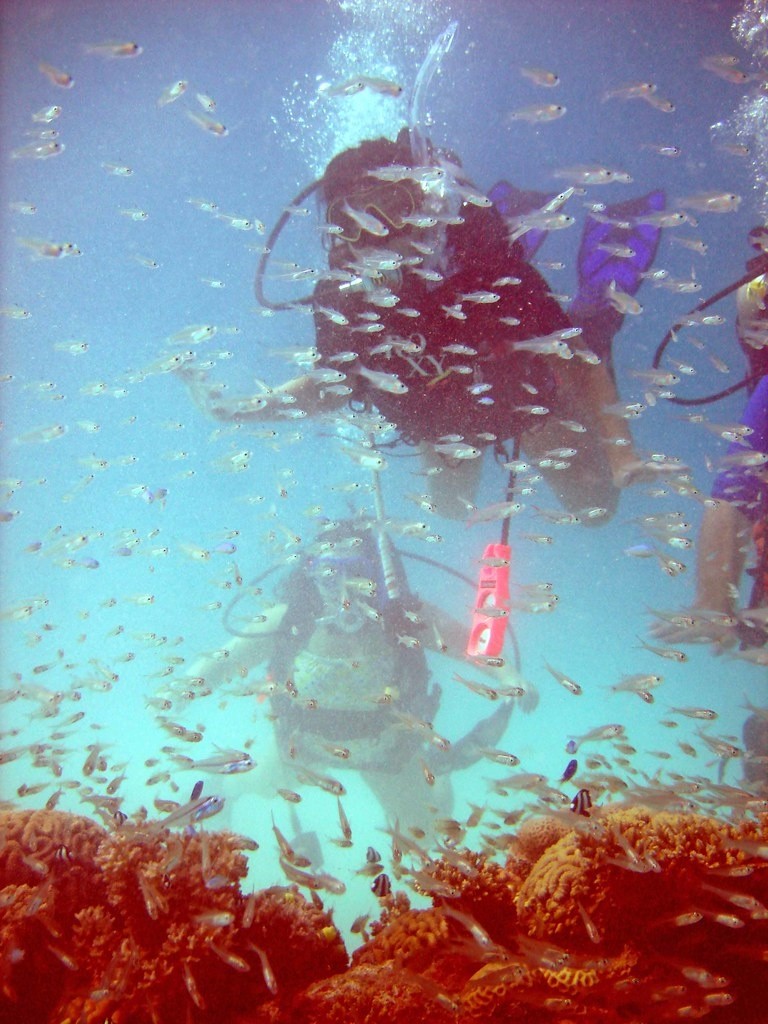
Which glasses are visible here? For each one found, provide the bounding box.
[326,180,417,243]
[311,557,373,588]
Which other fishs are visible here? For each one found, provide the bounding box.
[0,41,768,1024]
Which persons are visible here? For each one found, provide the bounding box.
[644,231,768,667]
[183,521,538,855]
[226,129,664,528]
